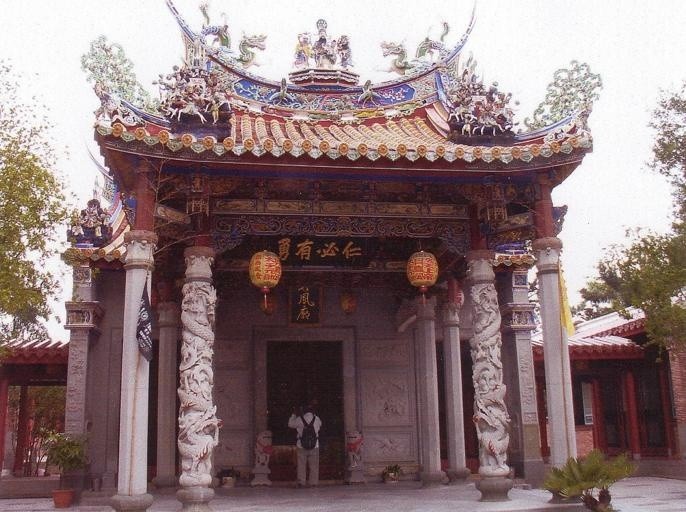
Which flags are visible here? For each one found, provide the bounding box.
[136,279,155,362]
[557,262,575,337]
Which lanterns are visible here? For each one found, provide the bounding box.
[406,252,438,308]
[249,250,283,309]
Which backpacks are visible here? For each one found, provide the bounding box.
[299,416,318,451]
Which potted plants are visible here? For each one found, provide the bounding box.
[382,464,401,484]
[42,432,91,508]
[544,449,639,512]
[215,468,241,488]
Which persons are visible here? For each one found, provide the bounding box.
[151,57,234,126]
[445,69,515,136]
[291,18,354,72]
[288,401,323,489]
